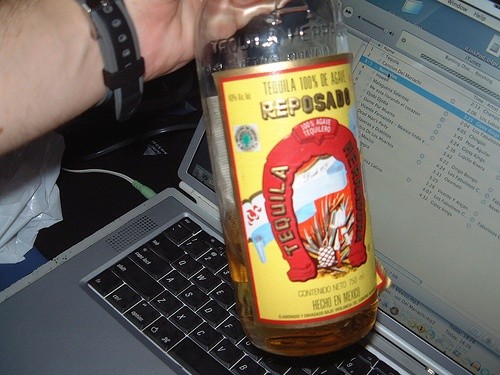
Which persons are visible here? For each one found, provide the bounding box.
[0,0,288,155]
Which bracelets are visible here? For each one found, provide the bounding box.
[81,0,146,118]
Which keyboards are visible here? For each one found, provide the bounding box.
[1,1,500,375]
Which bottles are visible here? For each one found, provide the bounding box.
[193,1,377,357]
